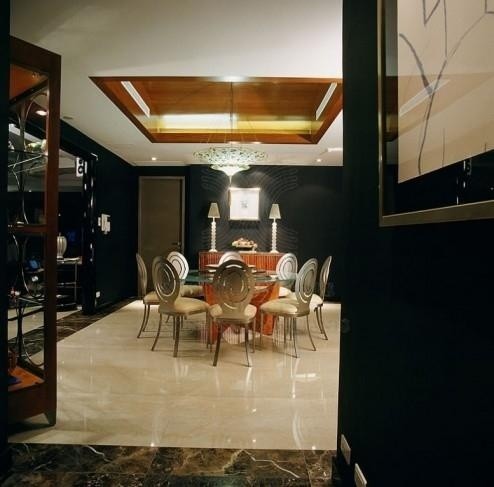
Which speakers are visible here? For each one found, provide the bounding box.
[75,158,88,178]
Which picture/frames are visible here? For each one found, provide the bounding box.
[227,187,261,221]
[376,0,494,228]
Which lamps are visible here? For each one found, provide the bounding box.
[268,203,281,253]
[208,202,220,252]
[192,82,267,186]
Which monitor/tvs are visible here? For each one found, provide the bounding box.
[27,259,40,269]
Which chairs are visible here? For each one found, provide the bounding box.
[134,251,332,366]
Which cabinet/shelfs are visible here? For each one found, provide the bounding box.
[6,32,61,424]
